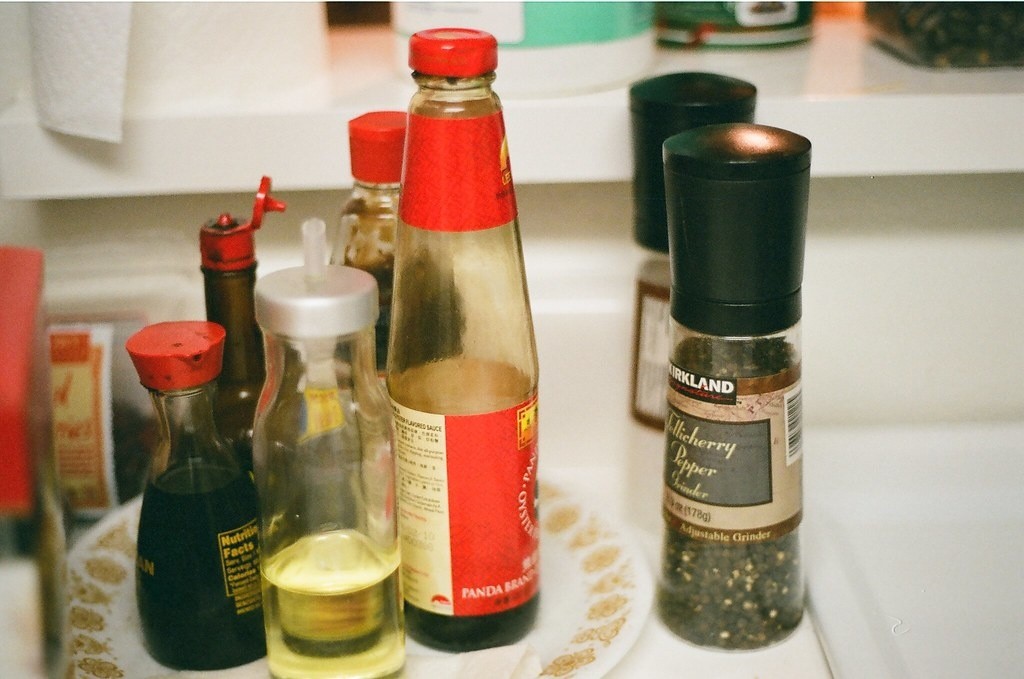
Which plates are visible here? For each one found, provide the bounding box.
[67,463,656,679]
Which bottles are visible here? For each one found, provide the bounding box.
[624,72,757,529]
[127,28,539,679]
[656,124,811,650]
[0,244,61,679]
